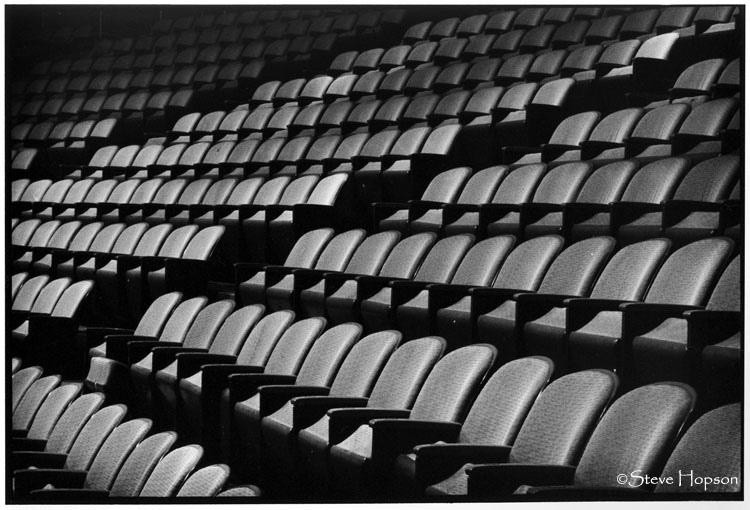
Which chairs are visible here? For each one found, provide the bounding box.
[6,4,746,503]
